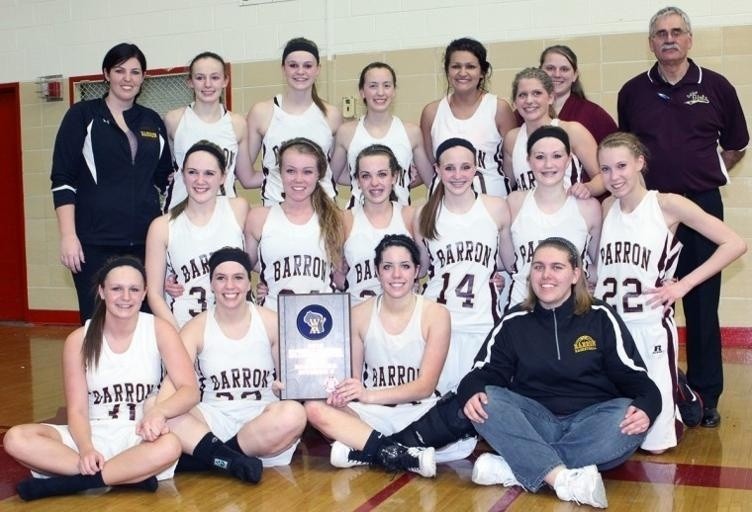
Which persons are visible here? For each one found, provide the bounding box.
[50,43,174,326]
[494,125,603,285]
[502,67,609,198]
[162,51,265,214]
[408,37,517,197]
[3,254,201,502]
[136,246,307,484]
[417,139,517,276]
[246,37,352,186]
[330,62,435,188]
[457,236,662,508]
[592,132,747,455]
[617,6,750,427]
[539,45,617,145]
[304,233,477,477]
[244,137,345,311]
[145,140,251,335]
[334,145,417,300]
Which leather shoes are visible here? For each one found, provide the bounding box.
[700,407,721,428]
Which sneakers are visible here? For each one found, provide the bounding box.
[212,445,262,483]
[553,464,608,509]
[380,442,437,478]
[471,454,528,492]
[676,367,704,429]
[329,441,375,468]
